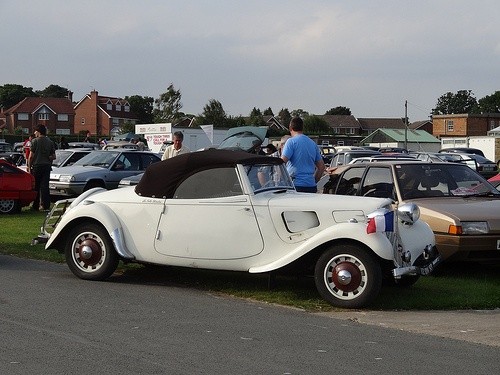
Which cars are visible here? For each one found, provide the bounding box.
[0,159,37,214]
[48,149,161,197]
[317,161,500,266]
[1,126,500,198]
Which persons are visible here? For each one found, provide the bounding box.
[280,116,325,193]
[256,135,292,187]
[27,124,55,210]
[23,134,34,171]
[160,131,189,162]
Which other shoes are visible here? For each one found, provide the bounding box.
[39,207,51,212]
[27,206,40,211]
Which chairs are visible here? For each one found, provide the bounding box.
[418,175,445,196]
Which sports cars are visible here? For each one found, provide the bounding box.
[29,148,441,310]
